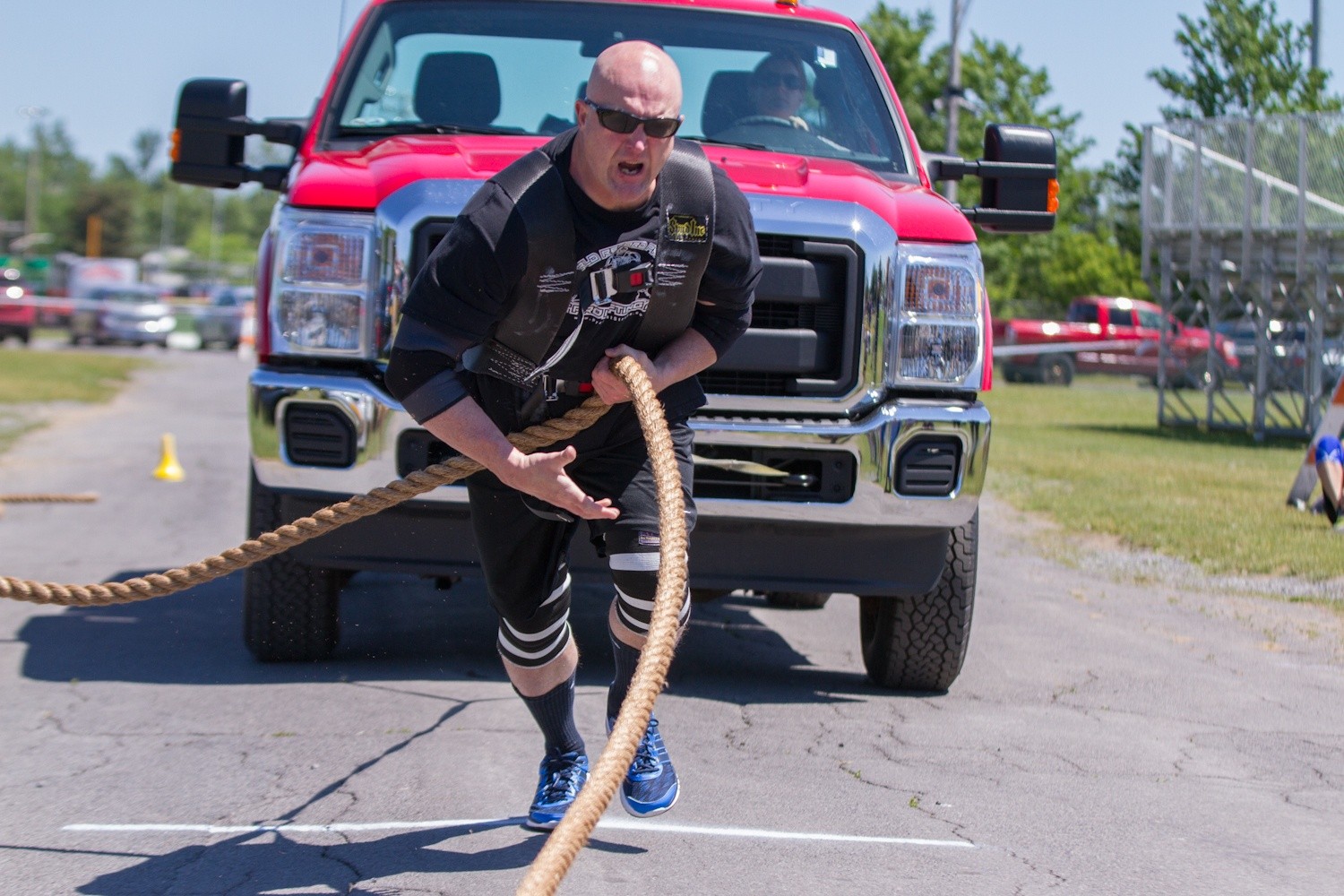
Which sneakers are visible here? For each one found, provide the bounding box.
[525,748,589,830]
[606,681,681,819]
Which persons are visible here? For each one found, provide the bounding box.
[750,49,818,135]
[1317,435,1344,524]
[387,40,764,828]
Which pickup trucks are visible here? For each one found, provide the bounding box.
[998,294,1242,394]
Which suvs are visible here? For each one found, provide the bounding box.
[165,0,1059,692]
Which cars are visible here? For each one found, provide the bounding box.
[1216,320,1340,392]
[0,245,256,352]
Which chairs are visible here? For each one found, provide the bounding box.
[700,69,756,137]
[413,50,523,132]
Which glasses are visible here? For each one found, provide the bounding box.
[757,71,802,89]
[584,97,681,137]
